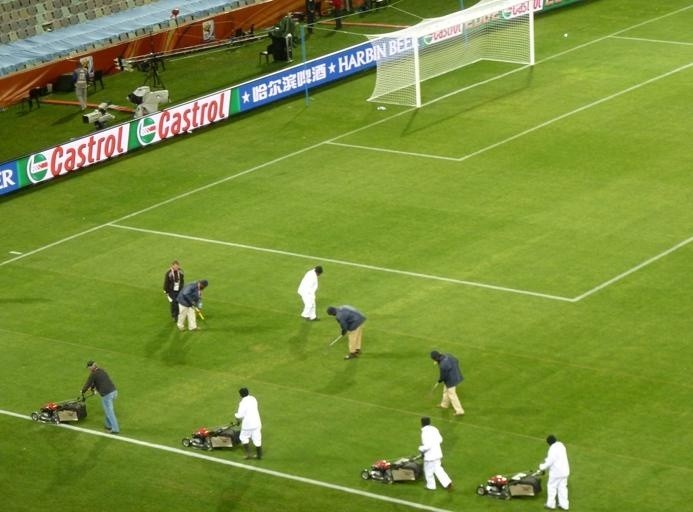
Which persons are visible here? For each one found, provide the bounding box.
[538,433,570,510]
[326,305,368,360]
[417,415,452,492]
[163,260,184,322]
[234,386,263,460]
[70,61,90,112]
[296,264,324,320]
[176,279,210,330]
[81,359,121,435]
[265,0,394,63]
[429,350,466,416]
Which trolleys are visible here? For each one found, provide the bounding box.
[31,389,97,424]
[476,468,545,501]
[182,420,240,452]
[359,451,424,484]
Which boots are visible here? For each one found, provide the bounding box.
[240,443,262,460]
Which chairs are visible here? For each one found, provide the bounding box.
[0,0,257,77]
[87,70,105,92]
[21,87,41,112]
[259,44,277,64]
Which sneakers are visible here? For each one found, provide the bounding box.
[435,401,464,418]
[344,350,362,360]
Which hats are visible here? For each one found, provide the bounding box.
[86,360,95,369]
